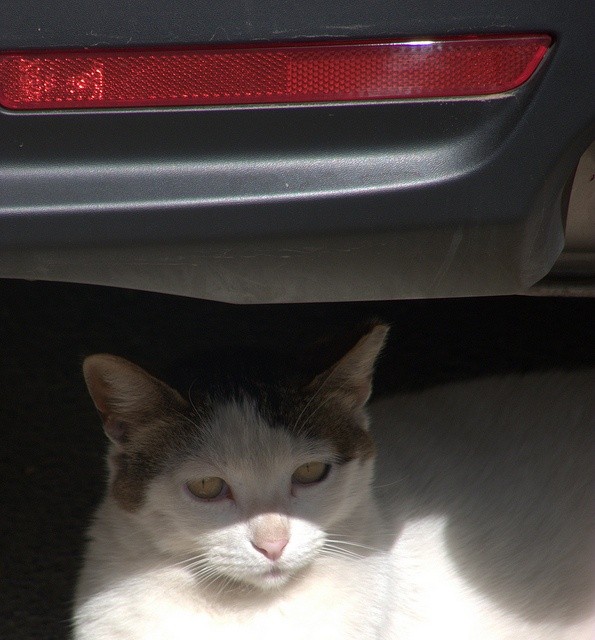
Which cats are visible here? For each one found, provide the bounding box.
[69,321,595,639]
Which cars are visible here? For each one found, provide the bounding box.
[0,0,595,321]
